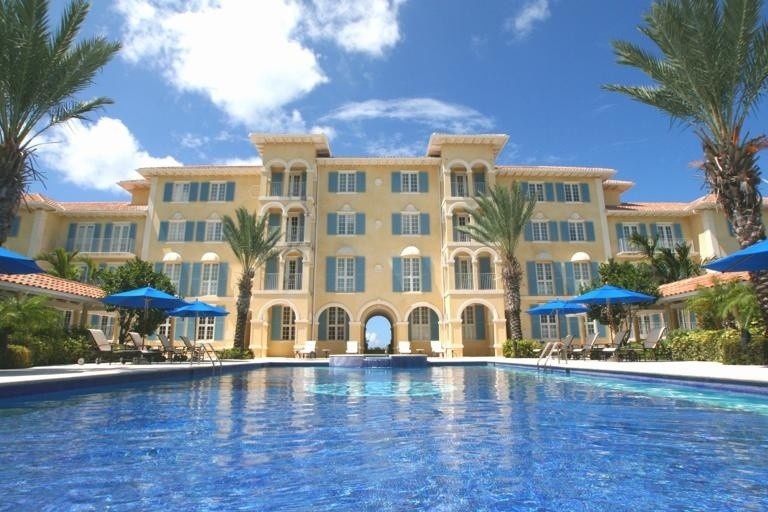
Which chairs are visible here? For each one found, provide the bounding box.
[345,340,359,354]
[398,338,445,358]
[550,326,670,362]
[83,328,206,365]
[298,339,317,358]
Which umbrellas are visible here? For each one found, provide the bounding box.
[165,297,231,361]
[564,280,658,362]
[526,297,593,357]
[0,246,48,276]
[100,283,194,364]
[699,238,768,274]
